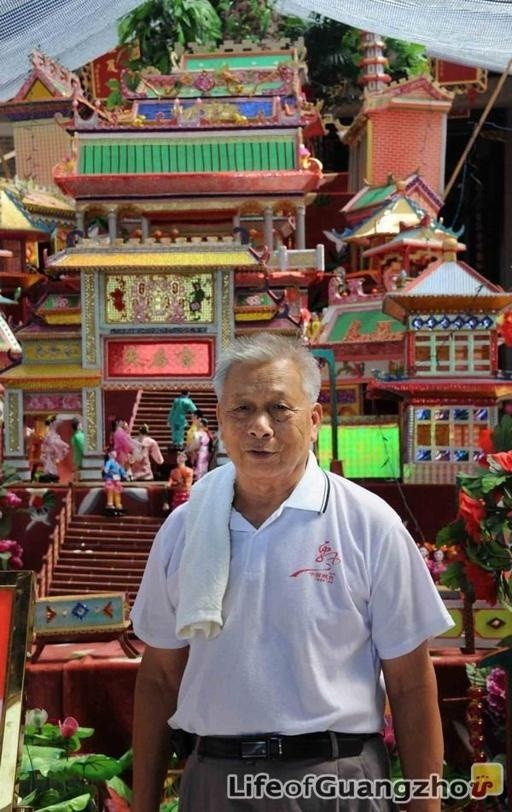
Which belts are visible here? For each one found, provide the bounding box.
[189,732,363,760]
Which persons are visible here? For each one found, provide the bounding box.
[26,390,214,518]
[125,329,456,810]
